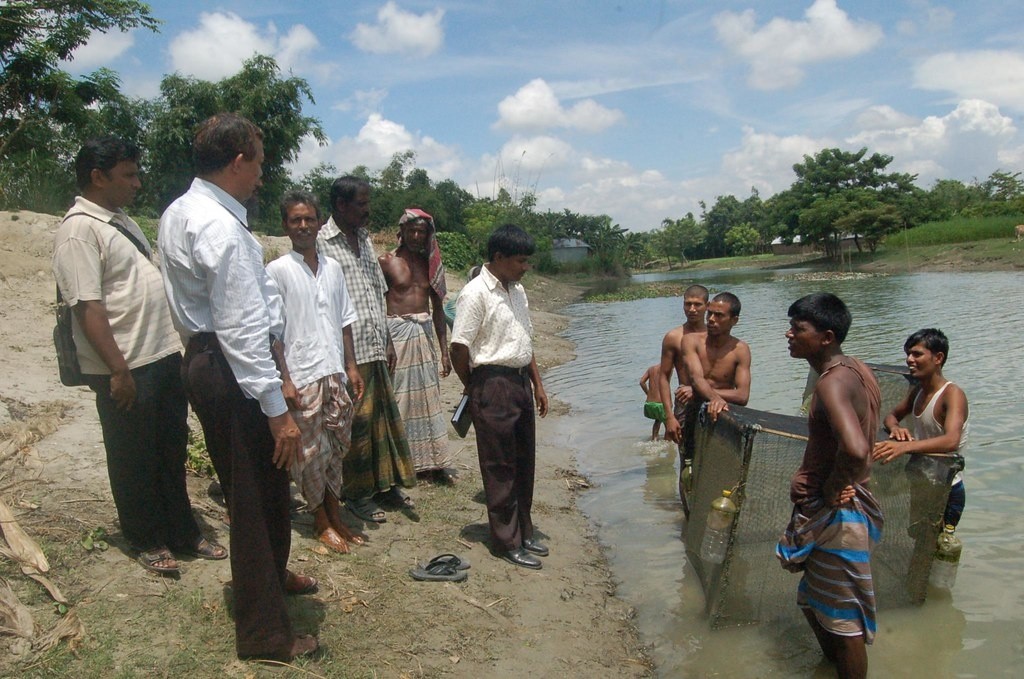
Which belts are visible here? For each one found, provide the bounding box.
[483,365,530,376]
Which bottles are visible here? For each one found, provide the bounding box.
[681,458,693,523]
[699,490,736,565]
[930,523,962,591]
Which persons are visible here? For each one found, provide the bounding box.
[50,137,229,573]
[873,328,968,540]
[261,188,369,556]
[157,112,323,665]
[639,363,673,442]
[448,224,550,570]
[773,290,884,679]
[675,292,751,463]
[376,207,455,482]
[658,285,711,522]
[315,173,418,521]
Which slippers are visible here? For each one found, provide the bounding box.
[416,554,470,569]
[352,500,386,523]
[381,486,415,510]
[409,560,467,582]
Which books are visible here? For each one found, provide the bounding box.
[451,394,472,438]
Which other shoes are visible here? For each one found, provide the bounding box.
[290,637,319,657]
[286,569,317,594]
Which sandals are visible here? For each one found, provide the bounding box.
[184,534,228,560]
[127,543,180,573]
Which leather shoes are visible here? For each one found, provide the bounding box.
[523,538,549,557]
[489,545,542,570]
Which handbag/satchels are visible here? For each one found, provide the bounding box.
[52,306,85,387]
